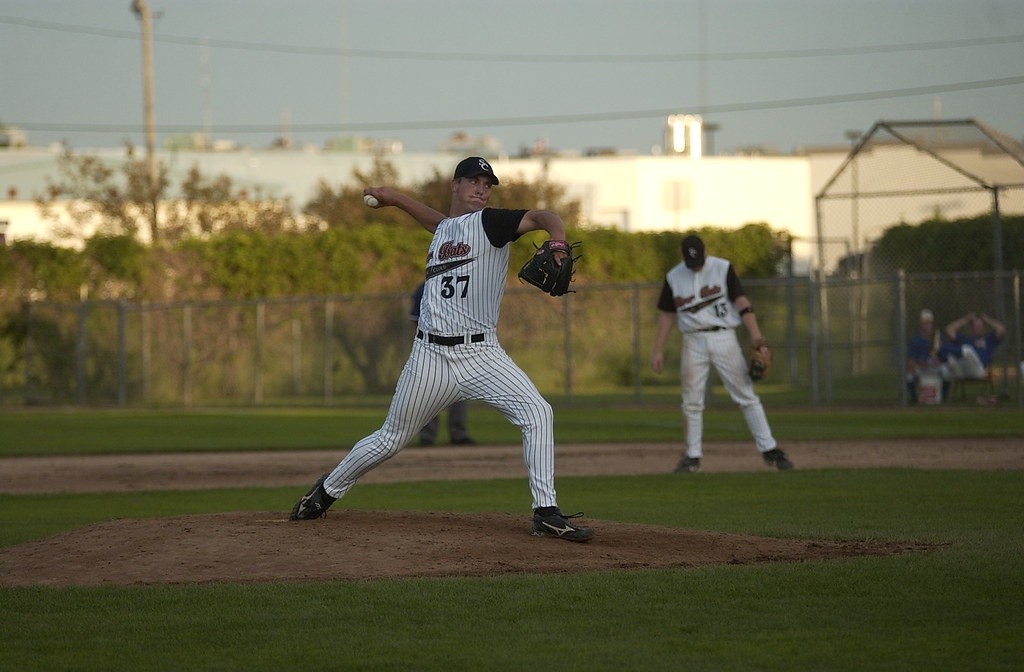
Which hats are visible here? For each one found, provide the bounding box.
[682,236,705,267]
[454,157,499,185]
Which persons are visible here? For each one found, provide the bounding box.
[907,310,1009,402]
[407,281,479,446]
[649,234,795,469]
[289,157,595,543]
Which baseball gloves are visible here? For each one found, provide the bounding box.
[517,238,584,298]
[748,336,772,383]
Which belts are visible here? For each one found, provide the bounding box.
[417,330,484,346]
[699,327,726,331]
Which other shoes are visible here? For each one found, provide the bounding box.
[763,447,793,470]
[674,455,700,474]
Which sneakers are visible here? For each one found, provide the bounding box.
[290,473,335,522]
[530,506,593,542]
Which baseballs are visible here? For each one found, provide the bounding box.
[363,194,379,208]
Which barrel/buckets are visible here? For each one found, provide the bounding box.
[918,376,942,403]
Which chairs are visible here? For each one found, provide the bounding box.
[954,354,995,401]
